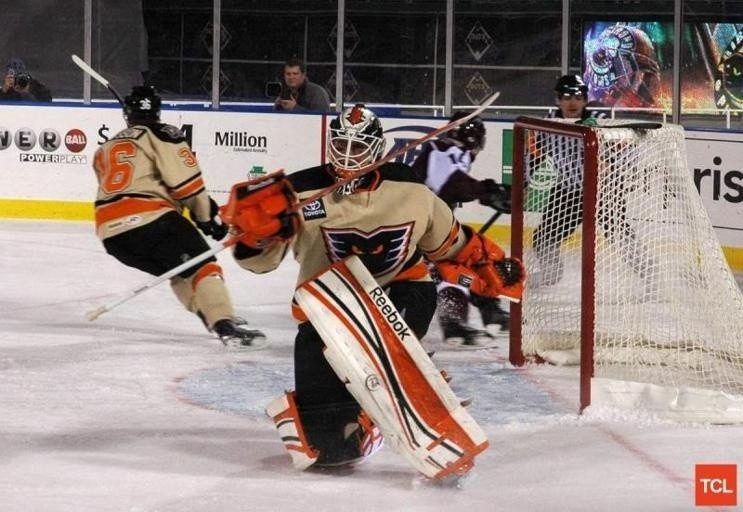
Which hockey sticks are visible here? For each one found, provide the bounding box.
[86,92,501,323]
[71,56,123,105]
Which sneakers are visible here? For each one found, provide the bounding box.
[480,308,510,330]
[442,321,496,346]
[196,308,265,347]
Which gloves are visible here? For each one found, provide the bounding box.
[190,196,227,240]
[480,178,512,215]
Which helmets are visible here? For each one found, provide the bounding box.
[122,85,161,120]
[327,102,386,197]
[555,74,589,91]
[447,109,487,158]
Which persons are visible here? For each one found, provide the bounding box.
[411,110,528,342]
[526,73,665,305]
[271,61,331,114]
[217,102,527,477]
[0,59,53,102]
[91,83,268,344]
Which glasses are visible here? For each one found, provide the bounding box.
[564,92,585,101]
